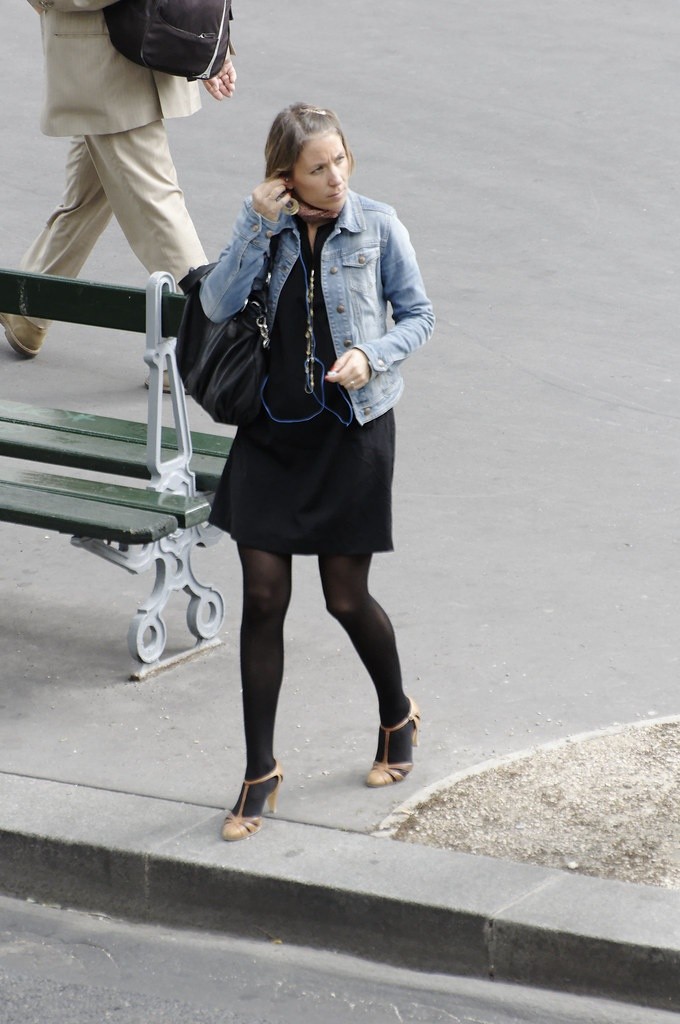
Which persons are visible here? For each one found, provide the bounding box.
[1,0,237,359]
[201,103,433,841]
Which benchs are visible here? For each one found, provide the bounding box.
[1,267,240,679]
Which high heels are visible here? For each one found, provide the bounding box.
[224,760,282,841]
[367,695,420,786]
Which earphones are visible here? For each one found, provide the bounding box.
[285,178,289,182]
[327,371,338,376]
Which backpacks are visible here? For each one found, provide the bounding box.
[103,0,236,82]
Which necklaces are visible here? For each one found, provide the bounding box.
[304,269,315,392]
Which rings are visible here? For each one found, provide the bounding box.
[269,194,276,201]
[350,381,354,388]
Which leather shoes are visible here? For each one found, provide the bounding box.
[145,370,191,394]
[0,312,44,357]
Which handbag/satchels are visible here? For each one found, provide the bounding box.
[173,233,279,425]
